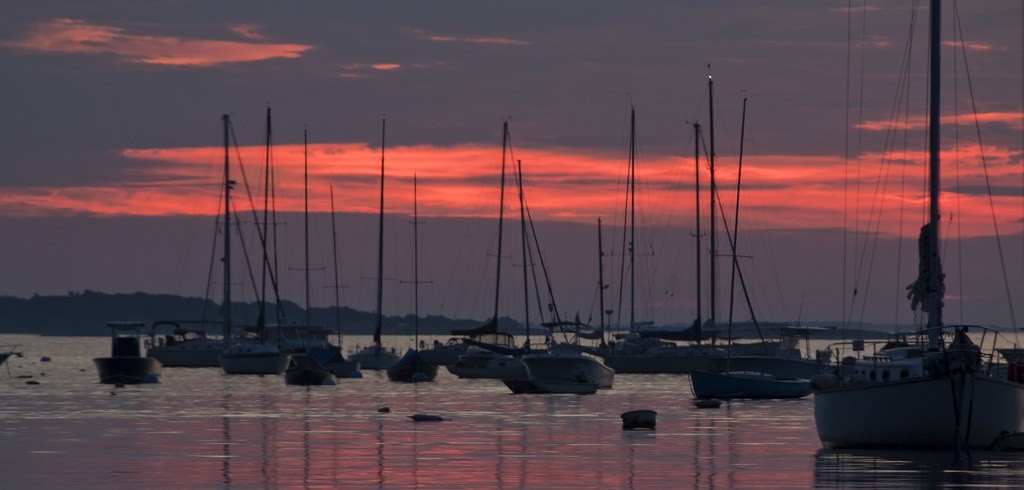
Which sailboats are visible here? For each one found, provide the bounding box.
[147,3,1024,459]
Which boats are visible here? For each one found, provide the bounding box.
[93,317,165,386]
